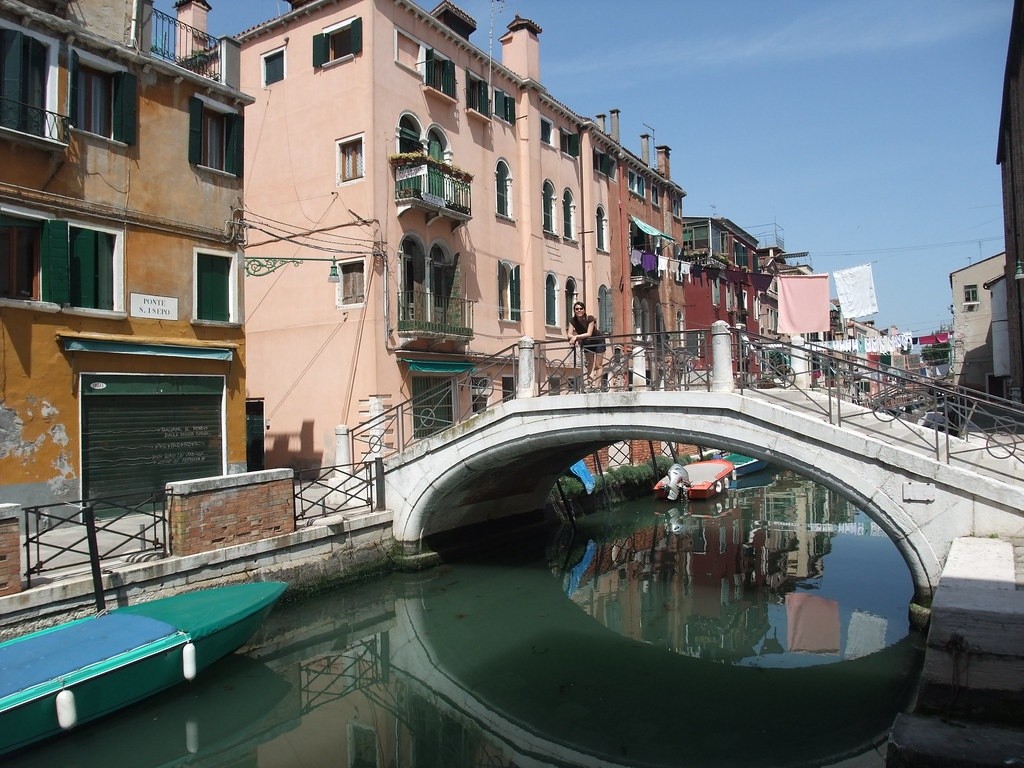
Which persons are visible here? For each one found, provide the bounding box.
[567,302,606,393]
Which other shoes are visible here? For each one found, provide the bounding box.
[594,388,600,393]
[583,386,594,393]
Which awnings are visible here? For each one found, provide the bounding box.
[631,215,676,255]
[403,358,476,373]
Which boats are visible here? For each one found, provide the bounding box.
[0,580,289,754]
[653,459,737,499]
[723,454,768,476]
[654,493,737,534]
[0,651,294,767]
[917,412,944,431]
[727,470,778,491]
